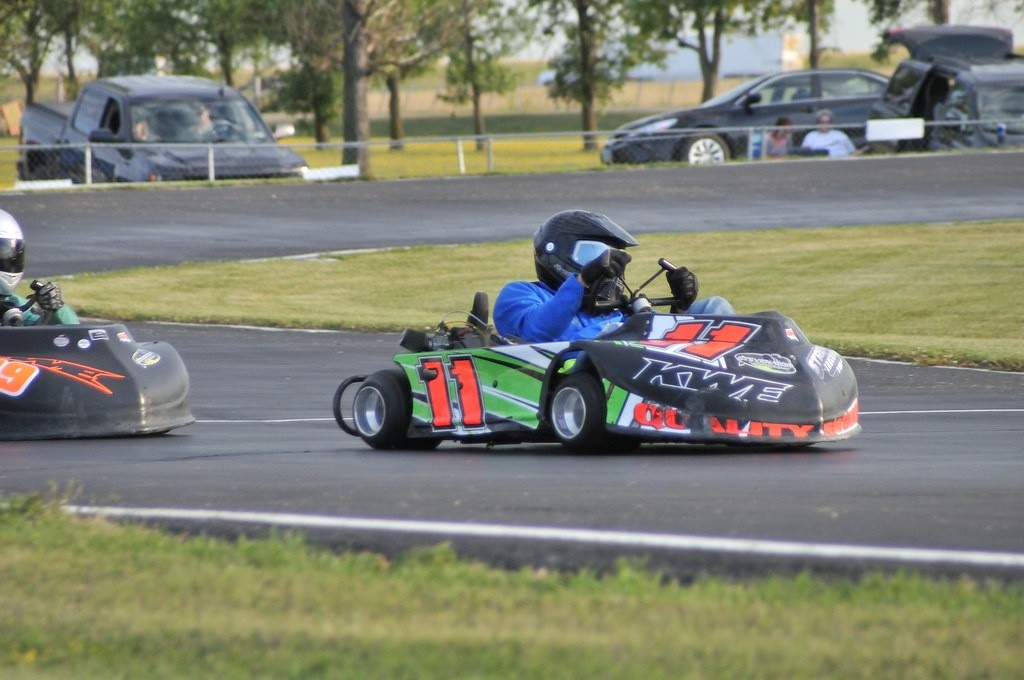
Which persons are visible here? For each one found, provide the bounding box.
[492,209,736,344]
[0,209,81,326]
[189,103,214,136]
[766,118,794,158]
[135,116,150,139]
[801,109,856,157]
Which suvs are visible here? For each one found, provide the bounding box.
[868,24,1024,153]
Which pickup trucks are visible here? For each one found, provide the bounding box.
[16,76,309,183]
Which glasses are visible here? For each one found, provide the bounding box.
[197,108,207,116]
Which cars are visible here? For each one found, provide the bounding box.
[601,67,890,166]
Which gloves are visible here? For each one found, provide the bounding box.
[665,266,699,312]
[581,248,633,287]
[36,281,65,312]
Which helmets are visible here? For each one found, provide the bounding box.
[532,209,640,311]
[0,208,25,298]
